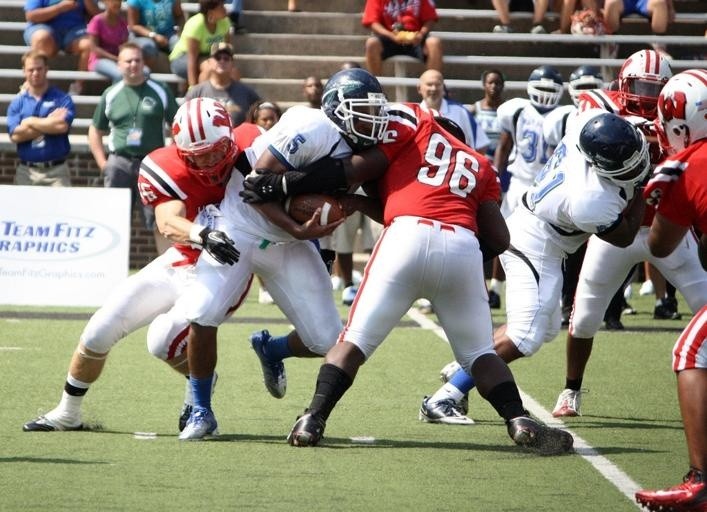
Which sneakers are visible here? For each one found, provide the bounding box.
[23,414,83,431]
[179,370,218,440]
[508,389,580,455]
[606,279,681,329]
[419,360,476,424]
[635,468,707,512]
[248,330,327,446]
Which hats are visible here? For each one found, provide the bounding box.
[210,41,233,55]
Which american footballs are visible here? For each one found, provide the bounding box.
[284,192,341,225]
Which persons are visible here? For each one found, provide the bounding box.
[22,50,707,511]
[362,0,442,78]
[492,0,677,36]
[7,0,265,232]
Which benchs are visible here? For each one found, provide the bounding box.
[0,1,707,157]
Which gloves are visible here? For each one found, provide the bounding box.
[239,168,285,204]
[199,226,241,265]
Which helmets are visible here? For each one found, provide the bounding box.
[527,50,707,187]
[171,97,239,187]
[320,67,389,152]
